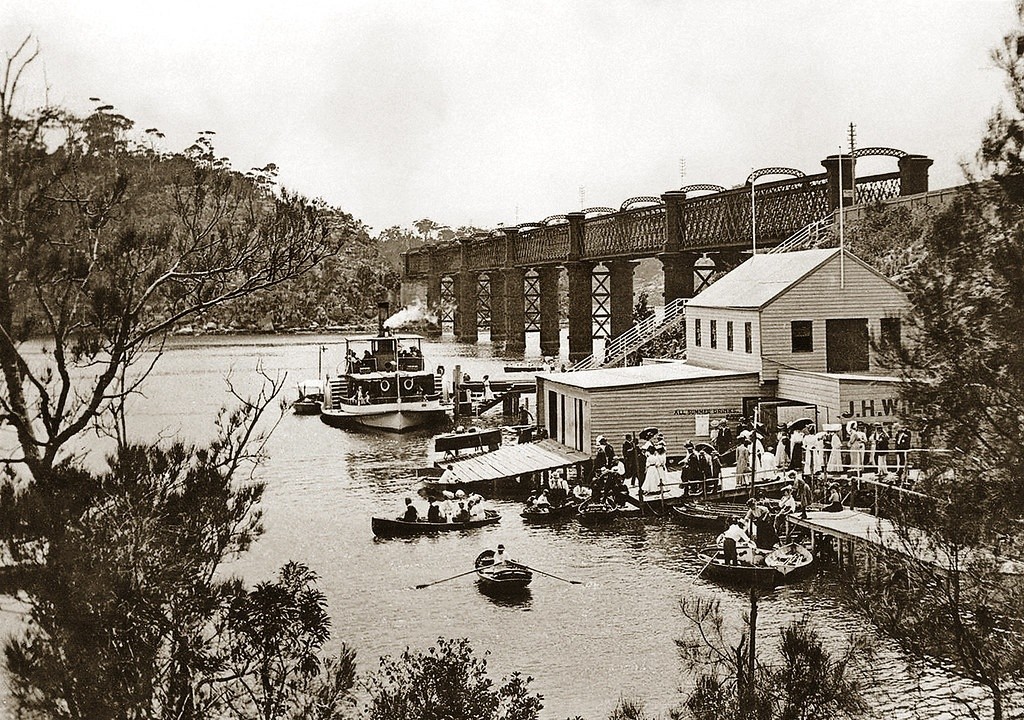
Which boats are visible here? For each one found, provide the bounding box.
[474,549,533,588]
[578,496,618,523]
[672,501,813,588]
[320,332,455,430]
[519,492,590,520]
[371,507,503,538]
[291,379,325,413]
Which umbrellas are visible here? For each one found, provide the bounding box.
[751,421,770,436]
[788,417,814,431]
[639,428,658,440]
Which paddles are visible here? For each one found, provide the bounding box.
[415,562,503,589]
[691,550,719,584]
[505,559,582,584]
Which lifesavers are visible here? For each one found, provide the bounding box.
[384,361,392,372]
[404,379,413,390]
[380,380,390,392]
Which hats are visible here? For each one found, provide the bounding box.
[776,422,787,429]
[611,455,620,462]
[496,544,505,550]
[719,418,728,423]
[787,470,797,476]
[710,420,719,427]
[805,424,814,431]
[738,518,748,526]
[828,481,840,490]
[455,490,465,498]
[746,498,756,506]
[736,435,747,439]
[442,490,454,499]
[781,484,792,491]
[857,423,864,428]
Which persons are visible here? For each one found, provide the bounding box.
[723,497,780,566]
[397,343,422,358]
[525,432,671,513]
[344,349,372,364]
[438,465,459,483]
[481,375,496,403]
[323,374,333,409]
[396,490,486,522]
[463,373,470,383]
[518,406,534,425]
[773,470,844,535]
[680,415,779,498]
[355,385,371,406]
[493,544,511,567]
[775,421,934,476]
[452,423,482,434]
